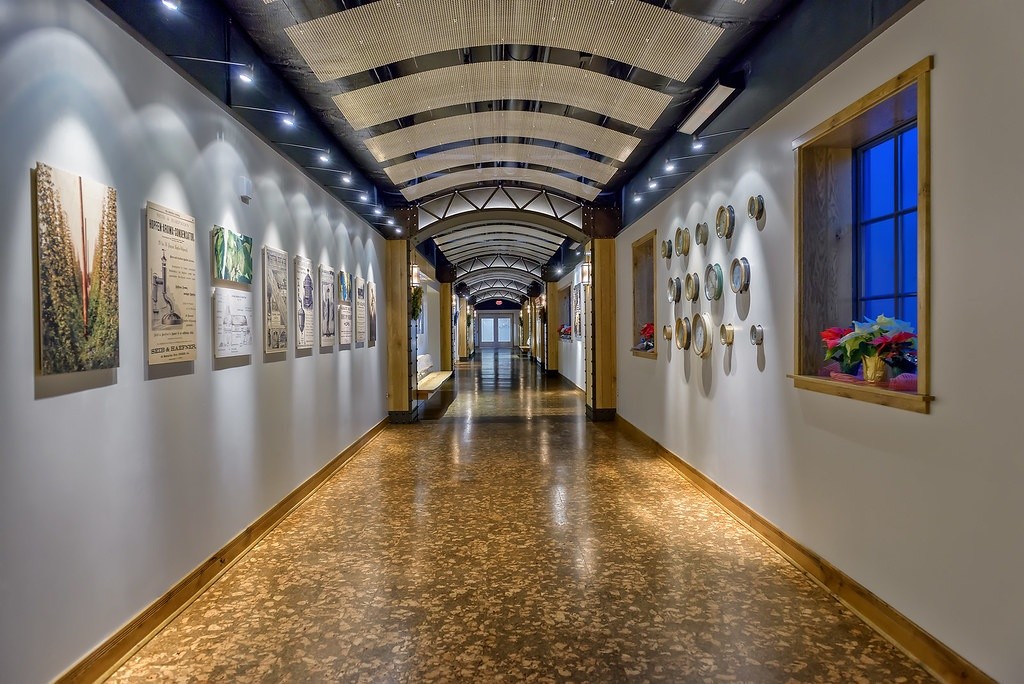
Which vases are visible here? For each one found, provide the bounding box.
[860,354,891,383]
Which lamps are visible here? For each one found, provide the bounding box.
[580,262,589,284]
[648,171,693,190]
[162,0,181,11]
[632,187,674,202]
[575,247,581,256]
[373,222,403,233]
[345,201,383,215]
[412,264,421,287]
[232,104,296,127]
[274,140,331,163]
[330,185,369,201]
[692,127,746,150]
[665,153,715,172]
[304,166,351,184]
[171,55,255,84]
[361,213,395,226]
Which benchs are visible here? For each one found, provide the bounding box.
[519,338,531,352]
[412,353,453,400]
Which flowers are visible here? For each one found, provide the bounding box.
[639,322,654,342]
[817,313,917,377]
[555,324,571,335]
